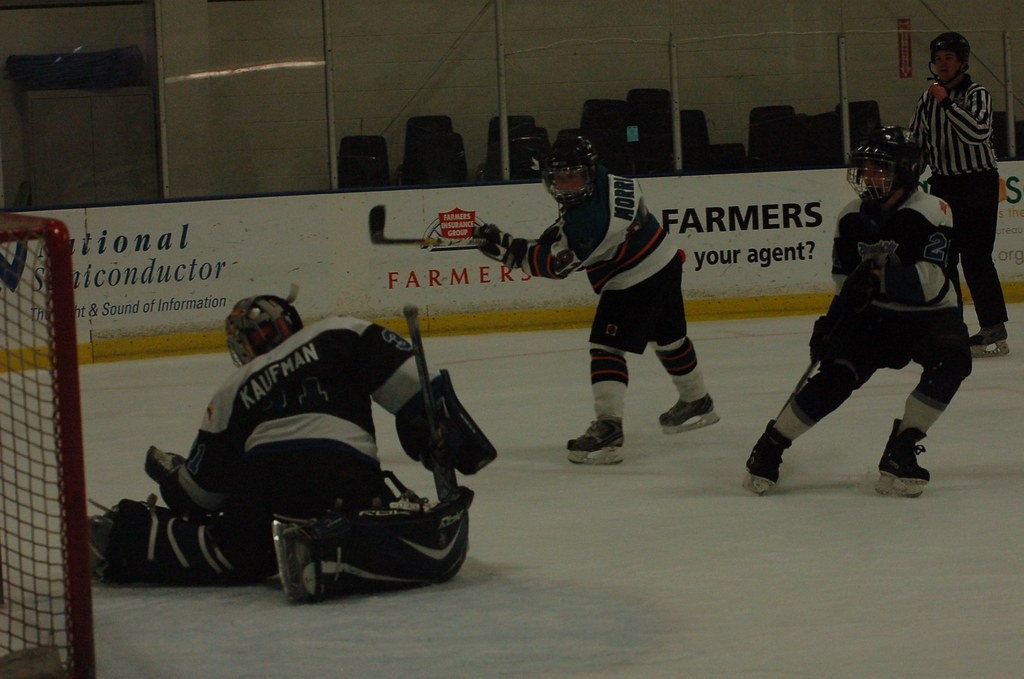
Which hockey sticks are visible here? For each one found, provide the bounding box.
[368,203,486,247]
[776,303,854,424]
[404,303,457,502]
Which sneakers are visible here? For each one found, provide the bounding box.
[87,513,107,585]
[271,519,332,602]
[659,393,720,436]
[875,419,930,498]
[745,420,792,496]
[969,321,1010,357]
[566,414,627,467]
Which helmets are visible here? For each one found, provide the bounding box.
[846,125,921,204]
[543,135,600,204]
[930,31,971,65]
[225,295,303,368]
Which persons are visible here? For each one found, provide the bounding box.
[744,124,972,483]
[84,294,474,604]
[471,135,713,451]
[908,32,1009,346]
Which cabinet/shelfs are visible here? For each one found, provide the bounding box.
[19,84,159,208]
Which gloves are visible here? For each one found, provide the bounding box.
[845,269,881,310]
[472,223,530,268]
[810,294,850,364]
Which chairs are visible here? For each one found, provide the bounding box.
[848,99,882,149]
[991,112,1017,161]
[748,104,794,170]
[708,143,747,174]
[681,109,710,174]
[1016,121,1024,161]
[798,111,843,170]
[508,125,552,181]
[483,114,535,180]
[836,106,868,155]
[416,132,471,187]
[338,134,391,191]
[402,116,454,187]
[762,113,808,170]
[562,129,637,178]
[581,99,652,177]
[627,88,674,177]
